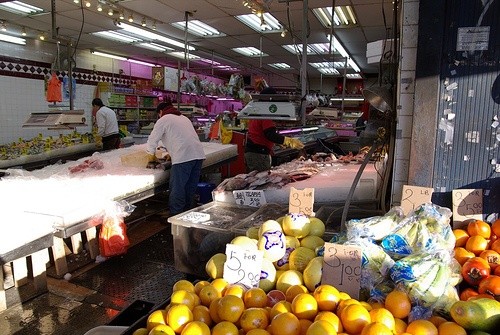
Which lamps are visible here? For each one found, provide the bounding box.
[0,0,364,79]
[361,84,395,115]
[204,39,220,99]
[174,9,198,93]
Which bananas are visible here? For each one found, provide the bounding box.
[344,205,464,312]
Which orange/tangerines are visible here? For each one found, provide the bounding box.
[133,278,470,335]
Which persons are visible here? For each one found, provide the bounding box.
[146,102,207,214]
[243,118,304,176]
[356,111,369,137]
[92,98,121,151]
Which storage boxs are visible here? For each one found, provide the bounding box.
[167,200,261,275]
[210,157,378,201]
[230,204,325,256]
